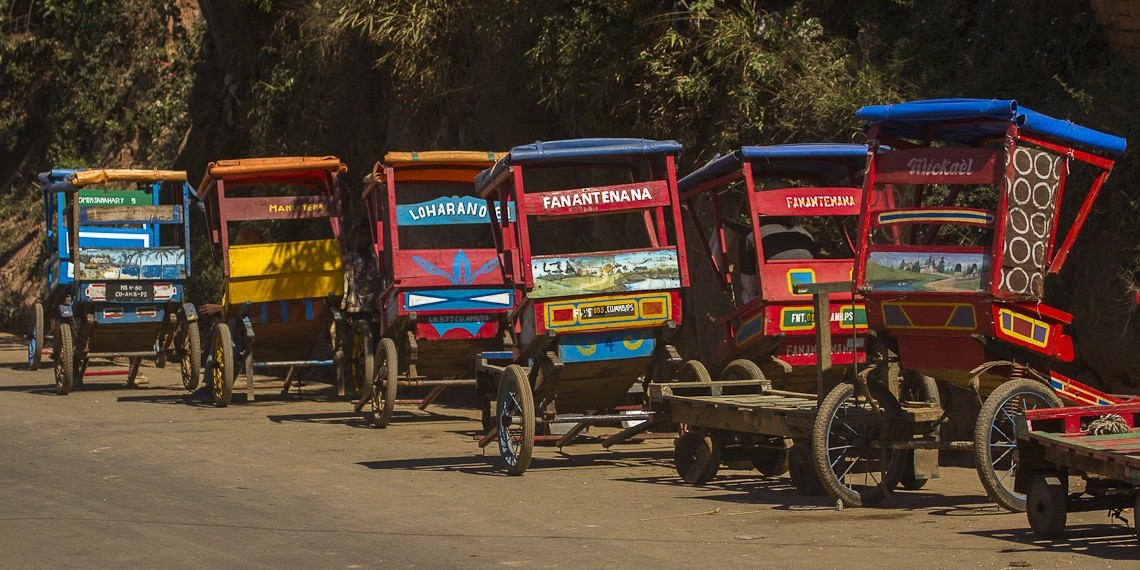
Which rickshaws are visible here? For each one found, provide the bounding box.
[194,155,358,408]
[663,94,1140,547]
[24,167,203,395]
[362,135,692,475]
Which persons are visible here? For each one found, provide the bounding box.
[188,225,264,402]
